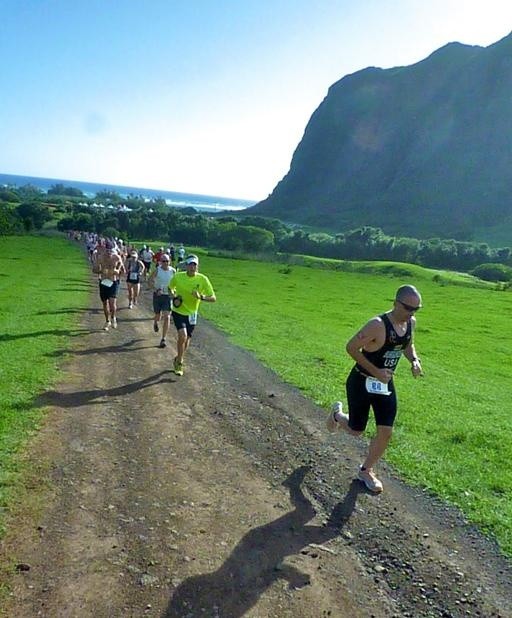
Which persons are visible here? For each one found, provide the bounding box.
[97,241,126,331]
[326,284,424,491]
[178,246,185,263]
[158,244,175,261]
[148,254,177,348]
[168,254,216,376]
[143,246,154,272]
[125,241,138,259]
[152,247,163,268]
[65,230,127,253]
[181,254,199,272]
[124,250,146,310]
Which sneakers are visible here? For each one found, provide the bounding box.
[356,465,385,492]
[175,360,184,376]
[154,322,159,331]
[160,339,166,347]
[103,321,111,331]
[128,303,132,308]
[326,401,343,434]
[111,317,117,328]
[134,300,137,305]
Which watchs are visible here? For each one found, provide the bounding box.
[409,357,420,363]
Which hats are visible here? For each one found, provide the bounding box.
[186,258,199,264]
[131,251,137,257]
[160,254,169,261]
[166,249,170,253]
[159,247,164,251]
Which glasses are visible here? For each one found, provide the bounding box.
[398,301,422,313]
[162,260,168,263]
